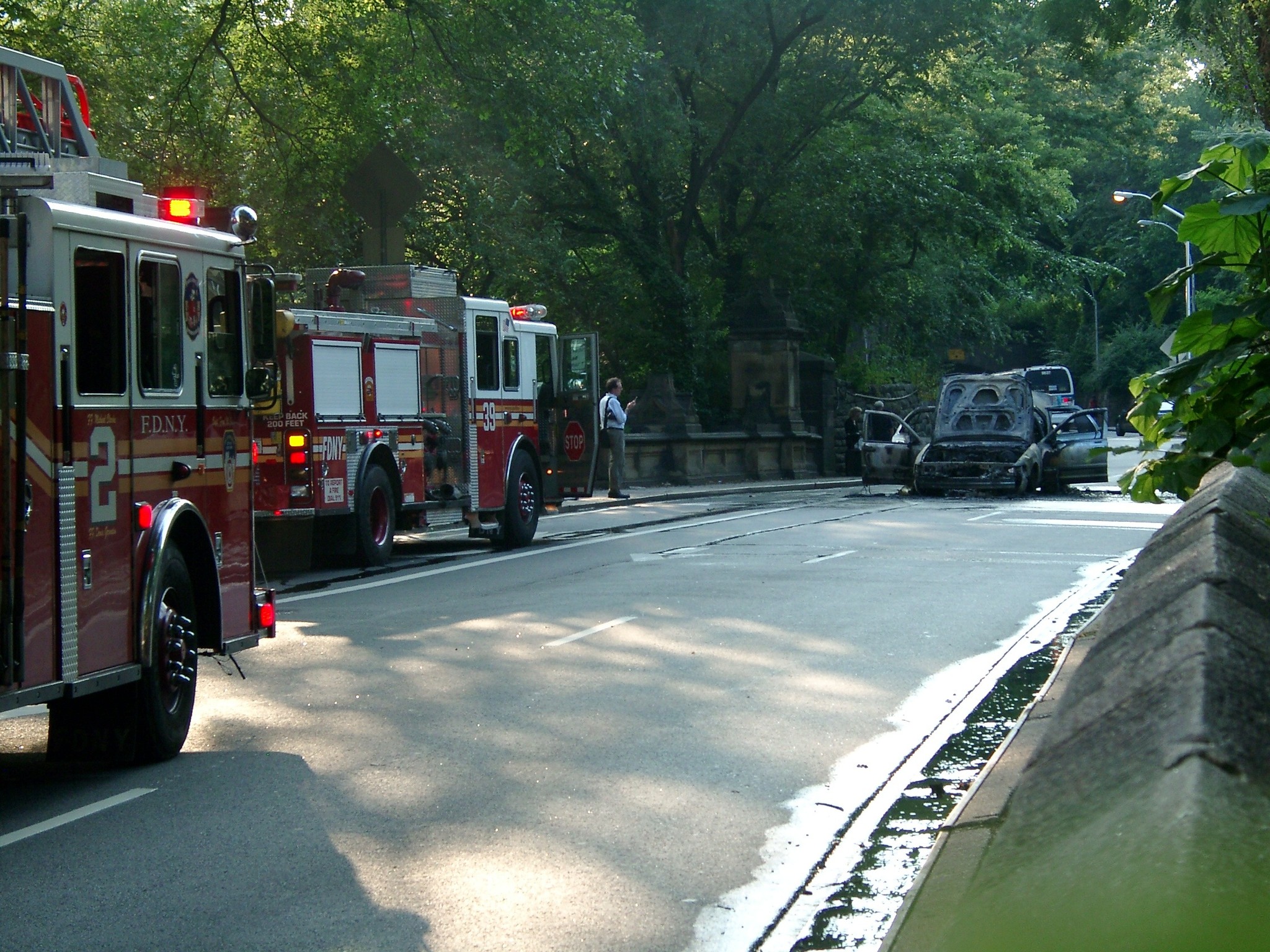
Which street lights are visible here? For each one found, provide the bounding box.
[1112,190,1195,395]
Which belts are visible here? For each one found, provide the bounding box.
[608,427,623,430]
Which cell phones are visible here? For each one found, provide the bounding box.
[634,396,638,402]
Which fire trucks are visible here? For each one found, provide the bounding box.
[238,258,604,572]
[0,42,296,777]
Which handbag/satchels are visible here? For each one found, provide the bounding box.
[858,437,863,451]
[607,410,616,420]
[599,428,611,449]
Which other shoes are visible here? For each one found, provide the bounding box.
[608,490,630,498]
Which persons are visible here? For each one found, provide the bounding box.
[599,377,636,498]
[869,400,894,442]
[844,407,862,476]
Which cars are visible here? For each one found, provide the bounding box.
[1115,397,1183,439]
[856,364,1111,497]
